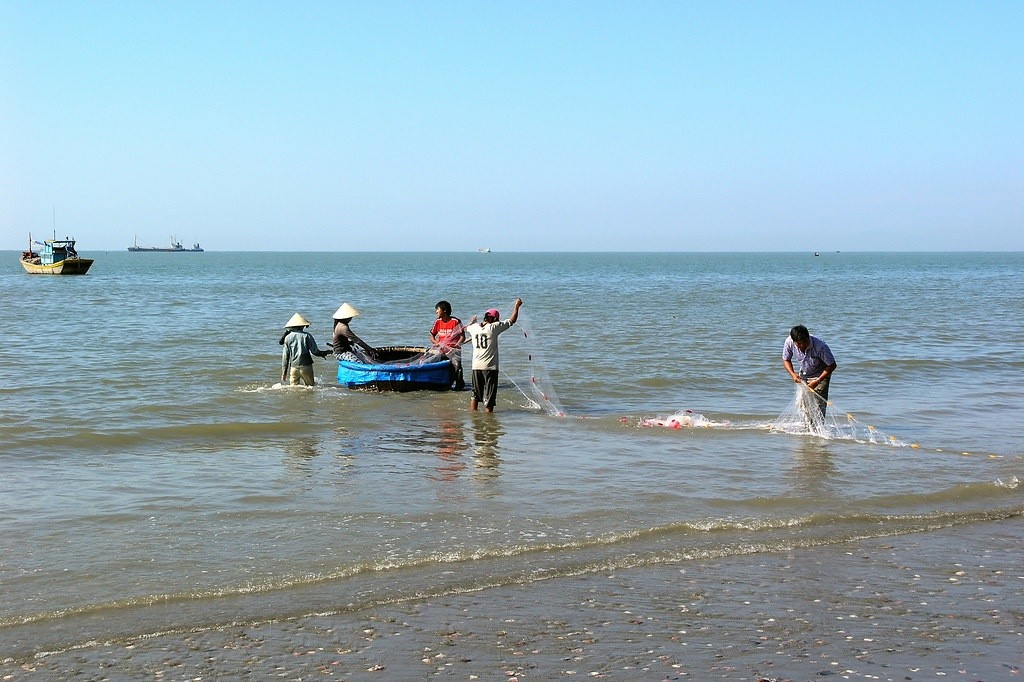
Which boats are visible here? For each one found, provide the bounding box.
[19,228,95,275]
[127,233,204,252]
[335,344,455,391]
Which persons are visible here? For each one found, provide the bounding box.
[280,313,333,386]
[331,302,378,364]
[429,300,465,394]
[466,298,522,413]
[782,325,837,426]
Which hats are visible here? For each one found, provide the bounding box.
[333,303,359,319]
[486,309,499,320]
[284,312,309,328]
